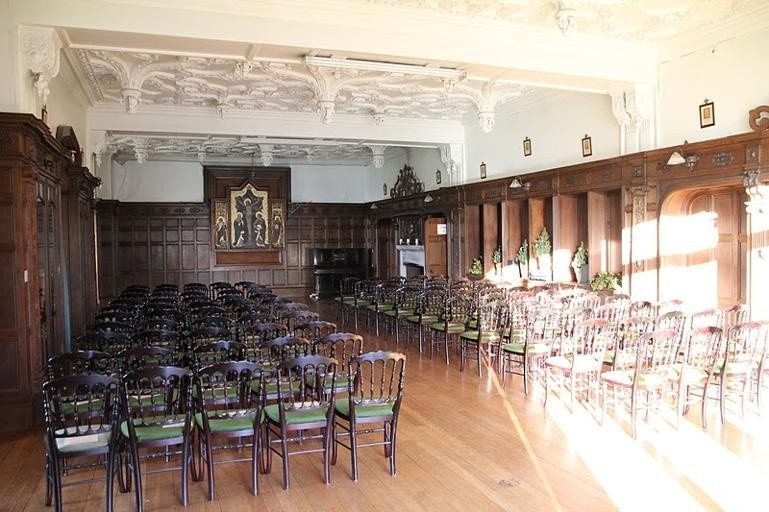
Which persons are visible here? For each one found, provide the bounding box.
[233,212,247,248]
[215,216,227,249]
[235,194,261,245]
[273,215,283,248]
[253,212,267,247]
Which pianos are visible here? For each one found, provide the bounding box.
[313,247,376,297]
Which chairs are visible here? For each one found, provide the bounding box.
[331,349,406,482]
[648,326,723,430]
[43,282,363,476]
[121,365,194,512]
[198,359,266,500]
[600,329,680,440]
[686,320,760,424]
[728,321,768,416]
[332,275,750,415]
[263,355,338,491]
[43,374,125,512]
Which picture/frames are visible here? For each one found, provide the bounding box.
[699,102,715,128]
[383,185,387,195]
[523,139,532,156]
[582,137,592,156]
[42,108,47,124]
[480,164,486,179]
[436,171,441,184]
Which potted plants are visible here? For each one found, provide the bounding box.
[465,224,621,295]
